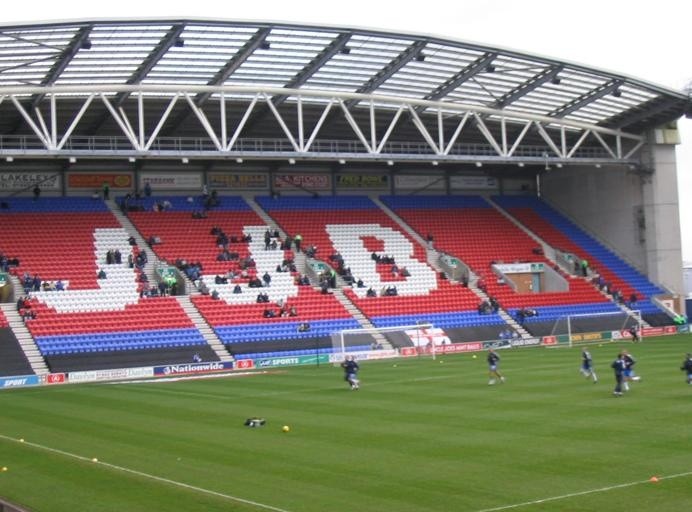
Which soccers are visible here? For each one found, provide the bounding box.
[3,466,8,472]
[92,458,98,463]
[282,425,290,432]
[20,438,25,444]
[472,354,476,357]
[650,476,658,483]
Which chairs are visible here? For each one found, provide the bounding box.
[0,193,668,377]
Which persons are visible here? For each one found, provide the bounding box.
[210,224,336,332]
[629,325,639,342]
[608,353,628,396]
[343,353,360,389]
[679,314,685,324]
[679,351,692,387]
[427,230,538,322]
[620,349,641,391]
[532,243,638,310]
[97,235,220,300]
[579,345,599,384]
[1,182,320,219]
[1,252,65,323]
[193,350,203,363]
[485,348,505,386]
[671,315,679,324]
[342,354,359,390]
[329,251,411,297]
